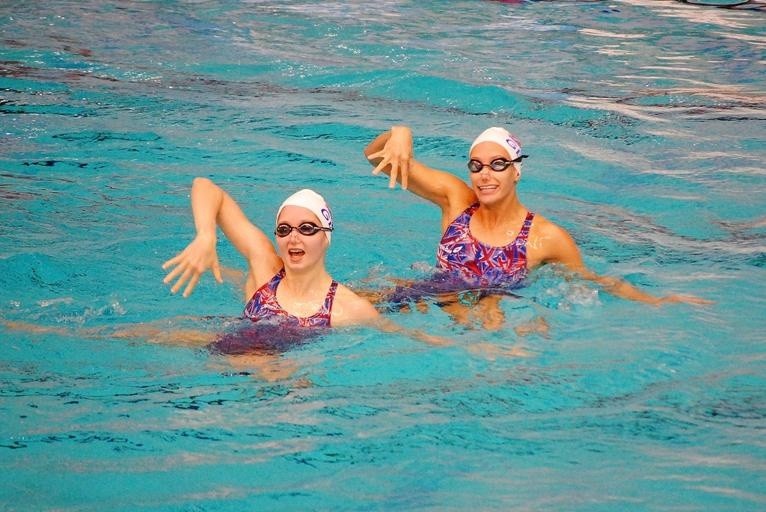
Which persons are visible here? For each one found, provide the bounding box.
[349,123,716,337]
[6,175,549,390]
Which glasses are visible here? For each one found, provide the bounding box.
[468,159,513,173]
[274,223,333,237]
